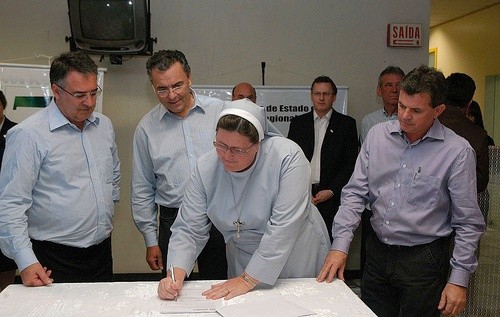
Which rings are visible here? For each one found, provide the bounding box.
[225,289,230,295]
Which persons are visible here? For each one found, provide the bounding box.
[157,99,331,300]
[0,90,18,292]
[360,62,494,279]
[316,66,487,317]
[233,82,256,103]
[131,49,229,281]
[0,51,122,286]
[287,75,358,242]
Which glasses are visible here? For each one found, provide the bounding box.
[312,91,334,97]
[55,83,102,100]
[212,134,254,155]
[152,76,187,96]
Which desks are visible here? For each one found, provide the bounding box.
[0,277,378,317]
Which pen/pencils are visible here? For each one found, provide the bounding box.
[414,166,421,180]
[170,264,177,301]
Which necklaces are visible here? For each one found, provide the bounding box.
[230,173,252,238]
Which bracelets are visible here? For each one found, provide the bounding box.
[328,189,334,197]
[241,274,256,288]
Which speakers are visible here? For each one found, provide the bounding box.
[67,0,150,56]
